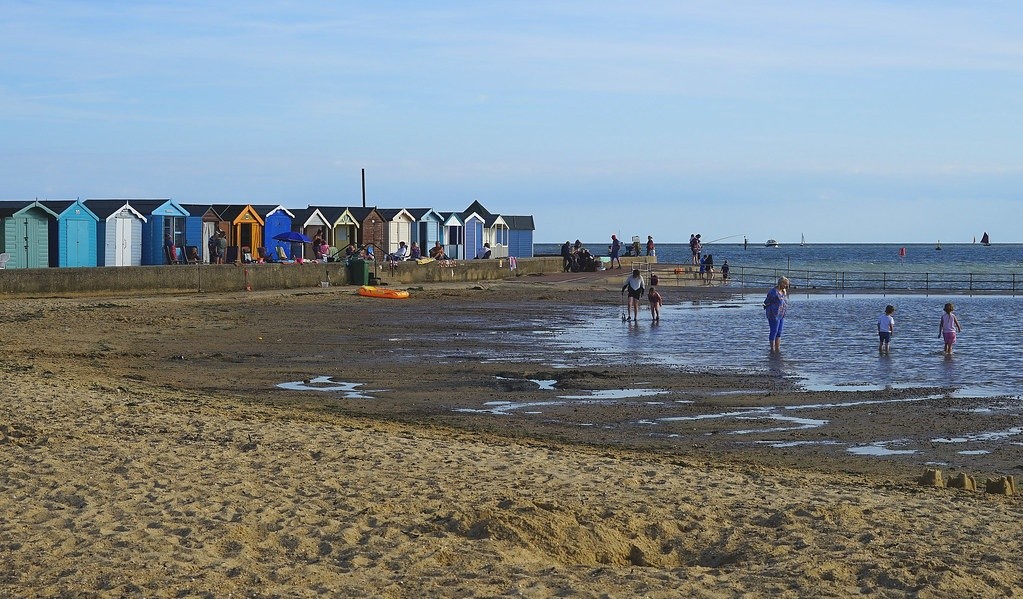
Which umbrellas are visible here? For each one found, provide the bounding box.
[272,231,311,259]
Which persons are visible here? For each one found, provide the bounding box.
[764,276,789,351]
[938,303,961,354]
[649,271,660,286]
[474,243,490,260]
[877,305,895,354]
[165,230,228,265]
[647,287,662,321]
[562,234,731,285]
[622,269,645,321]
[312,229,445,262]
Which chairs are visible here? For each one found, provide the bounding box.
[242,246,288,263]
[367,246,375,261]
[329,247,339,262]
[397,245,408,261]
[482,251,491,259]
[165,246,202,265]
[0,253,10,270]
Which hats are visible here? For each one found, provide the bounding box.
[696,234,700,238]
[567,241,571,246]
[611,235,616,238]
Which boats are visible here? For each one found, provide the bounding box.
[358,285,410,299]
[765,240,778,247]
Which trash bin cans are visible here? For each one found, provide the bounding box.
[226,246,238,263]
[350,258,369,285]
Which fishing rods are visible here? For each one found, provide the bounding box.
[684,233,747,263]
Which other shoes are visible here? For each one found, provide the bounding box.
[564,267,570,273]
[609,266,613,269]
[616,266,622,269]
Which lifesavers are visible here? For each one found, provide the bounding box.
[359,285,410,299]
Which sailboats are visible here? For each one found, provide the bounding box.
[973,236,976,244]
[980,232,992,246]
[936,240,942,250]
[800,233,805,246]
